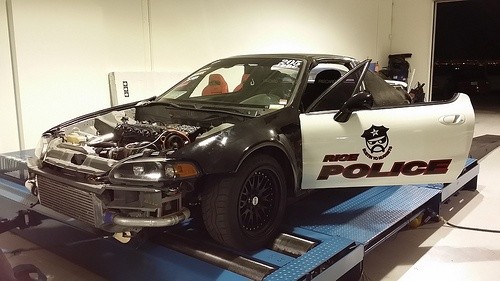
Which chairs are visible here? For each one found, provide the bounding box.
[301,68,348,111]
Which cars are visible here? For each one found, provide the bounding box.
[24,54,476,250]
